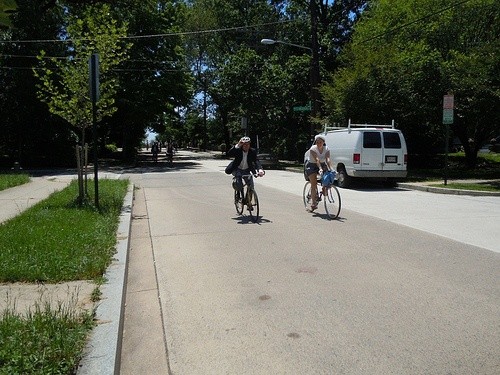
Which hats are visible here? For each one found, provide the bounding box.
[315,135,325,141]
[239,136,251,143]
[167,142,172,146]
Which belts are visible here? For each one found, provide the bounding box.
[238,168,249,171]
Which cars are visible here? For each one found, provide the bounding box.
[256,153,275,169]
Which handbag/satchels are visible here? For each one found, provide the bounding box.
[321,171,336,186]
[232,181,243,191]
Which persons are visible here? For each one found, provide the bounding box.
[304,134,338,209]
[151,142,159,161]
[224,136,264,211]
[166,143,175,161]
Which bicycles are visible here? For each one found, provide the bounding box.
[302,172,342,220]
[167,153,174,168]
[231,174,259,223]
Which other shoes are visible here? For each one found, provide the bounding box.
[235,194,239,201]
[247,206,253,210]
[310,199,316,207]
[169,160,171,162]
[322,188,328,196]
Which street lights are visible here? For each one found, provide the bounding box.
[261,39,320,114]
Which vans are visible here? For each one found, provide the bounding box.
[304,118,409,188]
